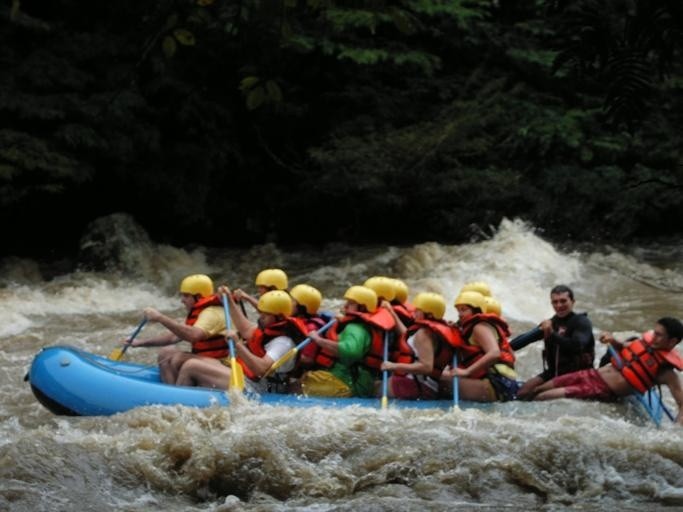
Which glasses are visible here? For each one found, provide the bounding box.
[550,299,570,303]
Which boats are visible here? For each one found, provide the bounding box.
[24,339,665,444]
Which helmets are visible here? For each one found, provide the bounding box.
[342,285,376,313]
[177,272,213,298]
[254,268,288,290]
[482,296,502,315]
[461,280,492,296]
[389,278,410,305]
[411,291,447,321]
[361,275,395,302]
[255,289,292,317]
[453,291,486,314]
[288,283,322,316]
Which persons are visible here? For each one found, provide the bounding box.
[512,285,594,393]
[534,317,682,426]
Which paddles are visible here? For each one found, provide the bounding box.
[263,319,336,377]
[108,318,147,361]
[222,293,245,395]
